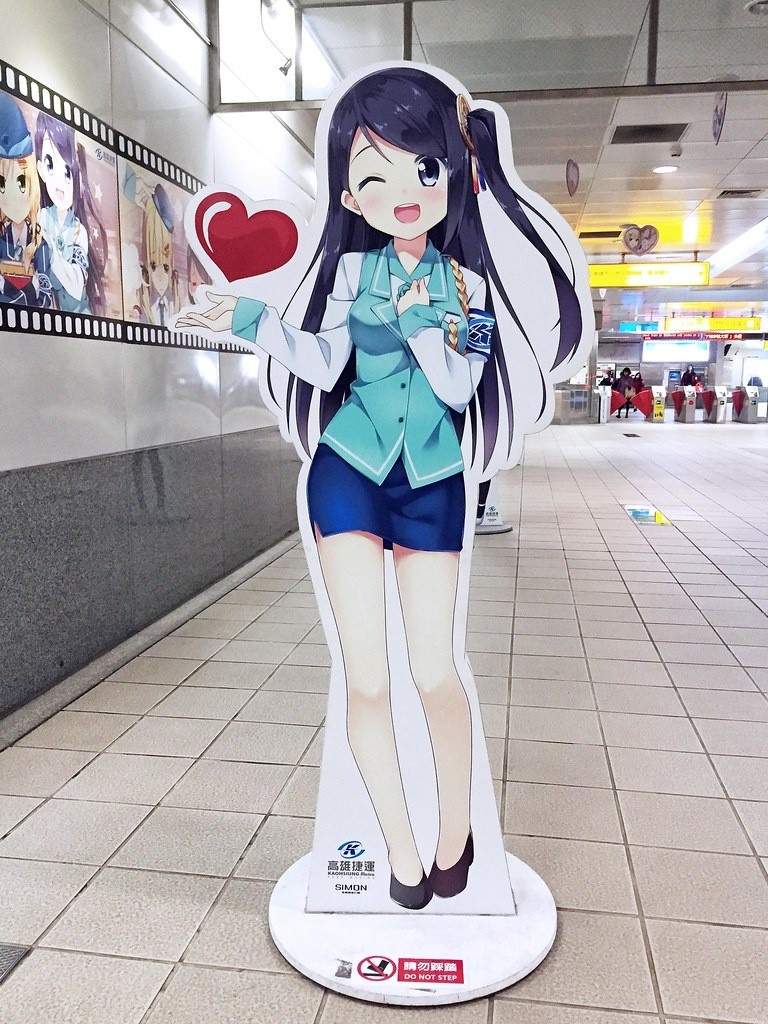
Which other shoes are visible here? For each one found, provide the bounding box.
[625,415,628,418]
[616,415,620,418]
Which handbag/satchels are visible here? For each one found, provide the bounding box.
[625,387,634,398]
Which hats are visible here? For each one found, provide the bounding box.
[607,371,613,375]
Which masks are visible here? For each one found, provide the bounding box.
[624,373,628,376]
[636,376,640,379]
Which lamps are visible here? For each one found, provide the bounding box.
[260,0,293,75]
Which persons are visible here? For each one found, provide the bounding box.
[680,364,698,386]
[598,367,644,418]
